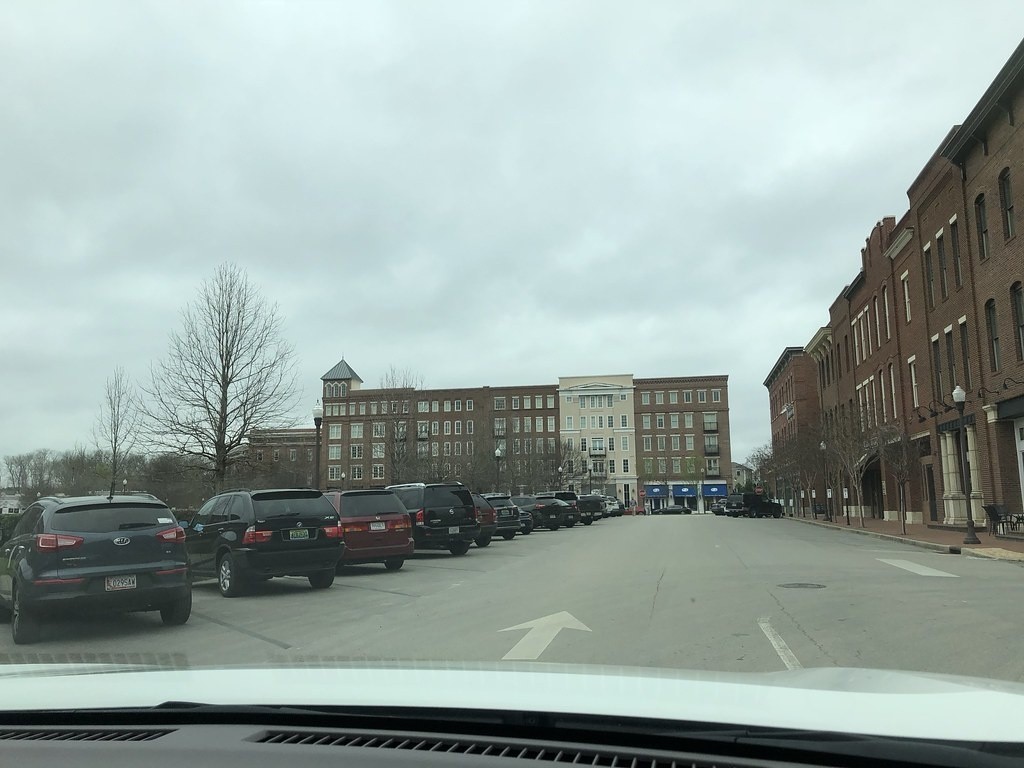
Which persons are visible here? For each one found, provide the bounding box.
[629,498,637,516]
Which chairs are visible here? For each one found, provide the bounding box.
[981,503,1024,536]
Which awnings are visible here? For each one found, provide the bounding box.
[644,485,668,498]
[673,484,697,497]
[702,484,729,497]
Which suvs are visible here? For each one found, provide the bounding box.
[0,491,193,646]
[723,491,783,518]
[321,486,416,571]
[383,480,482,556]
[183,487,346,597]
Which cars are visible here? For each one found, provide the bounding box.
[711,499,731,516]
[468,487,646,541]
[652,504,692,515]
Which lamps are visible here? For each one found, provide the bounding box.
[1003,378,1024,390]
[943,394,973,413]
[917,406,930,423]
[978,387,999,398]
[929,400,945,418]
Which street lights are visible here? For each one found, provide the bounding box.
[557,466,562,491]
[311,399,324,490]
[820,440,832,521]
[701,467,705,514]
[588,464,593,494]
[952,385,981,545]
[494,448,501,493]
